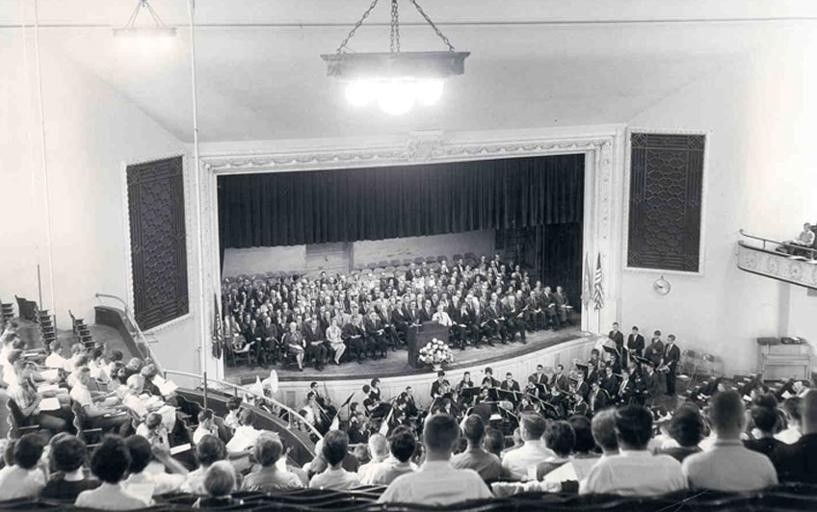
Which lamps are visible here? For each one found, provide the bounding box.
[317,1,472,115]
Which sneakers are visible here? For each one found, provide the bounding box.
[262,352,388,372]
[458,322,574,352]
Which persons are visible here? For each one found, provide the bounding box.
[222,251,575,372]
[0,321,815,512]
[783,223,815,255]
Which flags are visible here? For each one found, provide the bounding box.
[580,254,593,310]
[592,254,605,310]
[211,294,224,359]
